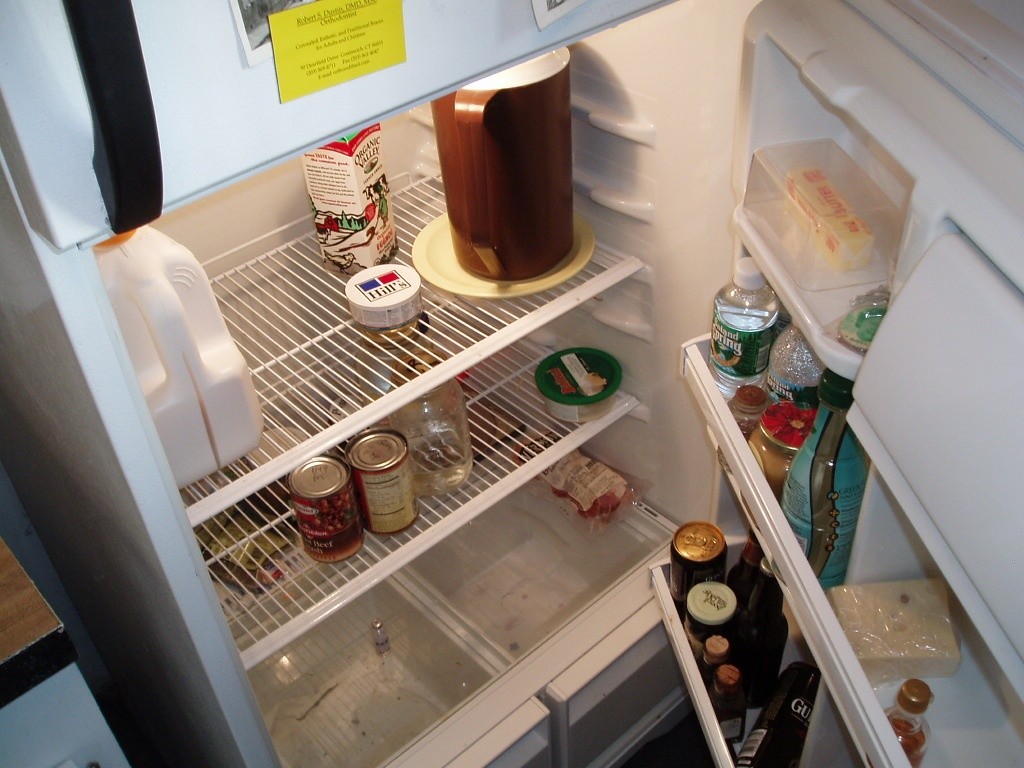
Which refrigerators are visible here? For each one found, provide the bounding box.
[0,0,1024,766]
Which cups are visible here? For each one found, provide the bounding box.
[434,46,574,288]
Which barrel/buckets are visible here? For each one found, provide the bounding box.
[92,223,264,486]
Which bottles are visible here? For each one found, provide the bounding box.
[351,315,473,494]
[697,635,742,716]
[706,256,872,593]
[727,524,788,709]
[370,616,392,655]
[866,675,934,765]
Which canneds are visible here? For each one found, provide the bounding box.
[284,385,823,768]
